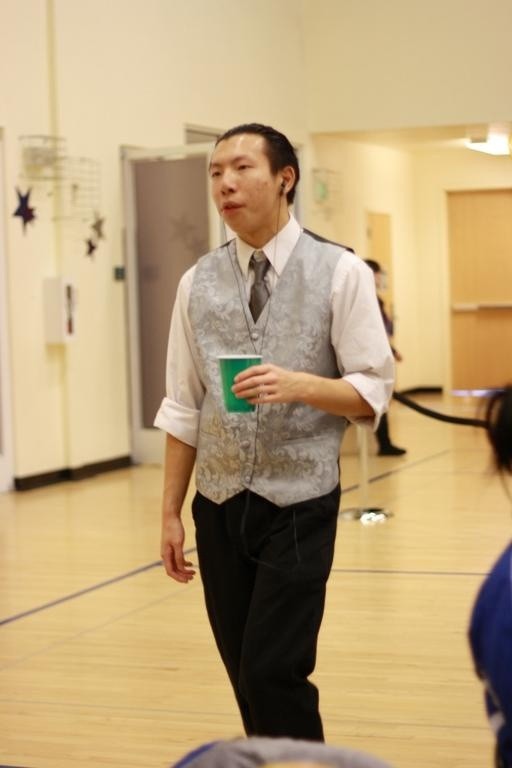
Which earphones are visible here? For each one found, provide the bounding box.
[279,180,287,195]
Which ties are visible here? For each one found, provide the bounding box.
[246,252,271,326]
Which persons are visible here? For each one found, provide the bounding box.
[463,379,510,767]
[355,257,410,458]
[151,121,401,751]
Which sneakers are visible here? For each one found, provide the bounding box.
[376,446,407,457]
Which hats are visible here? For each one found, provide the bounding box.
[364,260,386,275]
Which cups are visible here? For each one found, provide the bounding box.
[218,353,263,414]
